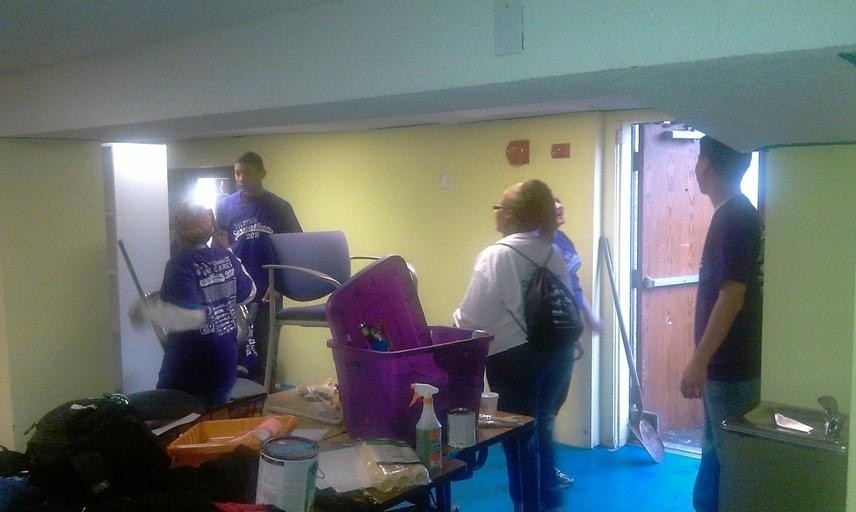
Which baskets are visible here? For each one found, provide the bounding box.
[166,414,297,469]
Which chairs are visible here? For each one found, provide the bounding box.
[259,231,419,428]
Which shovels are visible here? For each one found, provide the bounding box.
[601,238,665,463]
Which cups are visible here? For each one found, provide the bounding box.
[478,392,499,428]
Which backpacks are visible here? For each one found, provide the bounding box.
[493,242,585,353]
[0,393,172,510]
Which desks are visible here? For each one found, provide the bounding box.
[169,387,536,511]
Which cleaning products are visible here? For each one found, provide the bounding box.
[408,382,442,478]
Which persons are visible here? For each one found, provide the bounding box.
[126,202,256,408]
[207,153,302,418]
[450,177,602,512]
[678,136,765,512]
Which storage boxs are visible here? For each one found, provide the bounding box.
[324,254,494,449]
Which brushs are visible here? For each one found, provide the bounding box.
[478,413,524,428]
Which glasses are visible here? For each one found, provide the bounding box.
[492,204,513,212]
